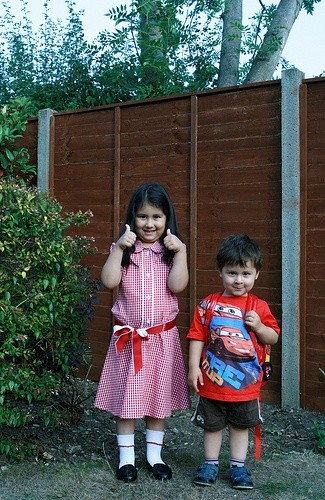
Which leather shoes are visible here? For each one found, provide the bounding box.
[117,457,137,483]
[145,458,173,482]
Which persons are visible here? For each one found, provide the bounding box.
[93,182,189,483]
[184,233,281,490]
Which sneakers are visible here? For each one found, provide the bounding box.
[193,460,220,487]
[229,465,254,490]
